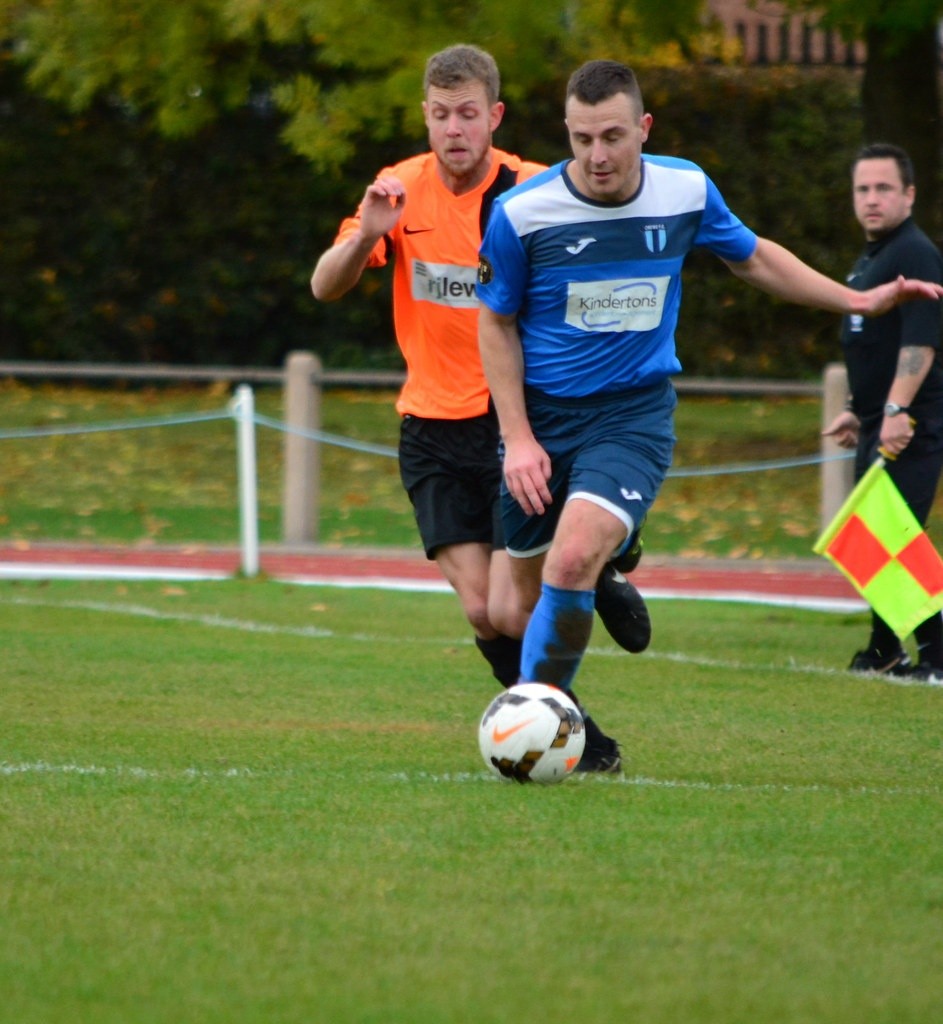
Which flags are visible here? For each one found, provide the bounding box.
[821,469,943,642]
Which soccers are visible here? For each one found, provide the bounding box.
[474,680,585,786]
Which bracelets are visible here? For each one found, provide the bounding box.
[844,399,853,413]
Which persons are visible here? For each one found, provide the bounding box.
[473,61,943,692]
[309,45,652,774]
[821,143,943,682]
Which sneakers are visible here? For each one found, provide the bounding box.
[847,646,910,676]
[905,662,943,683]
[615,540,644,572]
[576,710,622,774]
[593,564,652,654]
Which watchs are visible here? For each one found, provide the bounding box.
[884,403,909,417]
